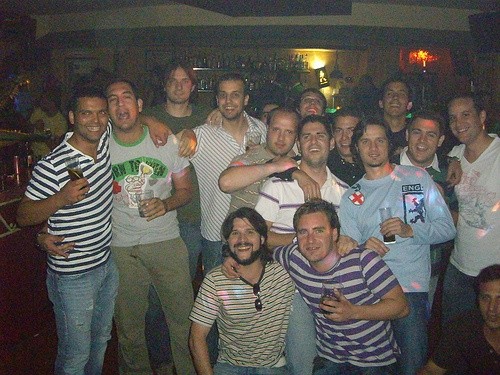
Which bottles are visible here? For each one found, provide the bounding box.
[0,141,34,192]
[173,51,309,117]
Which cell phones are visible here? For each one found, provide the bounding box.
[383,234,395,245]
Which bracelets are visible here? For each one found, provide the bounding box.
[33,232,45,248]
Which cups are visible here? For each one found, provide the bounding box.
[135,190,156,218]
[245,131,261,149]
[65,156,88,191]
[380,207,396,245]
[319,280,344,315]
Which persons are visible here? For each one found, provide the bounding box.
[35,80,195,375]
[339,116,457,375]
[298,88,326,118]
[189,208,357,375]
[16,80,172,375]
[0,58,500,375]
[221,198,411,375]
[140,60,215,375]
[379,76,410,165]
[411,264,500,375]
[178,73,266,367]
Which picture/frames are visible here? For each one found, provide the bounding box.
[146,50,174,72]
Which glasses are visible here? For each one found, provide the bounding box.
[253,283,263,312]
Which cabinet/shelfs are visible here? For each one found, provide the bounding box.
[191,67,311,93]
